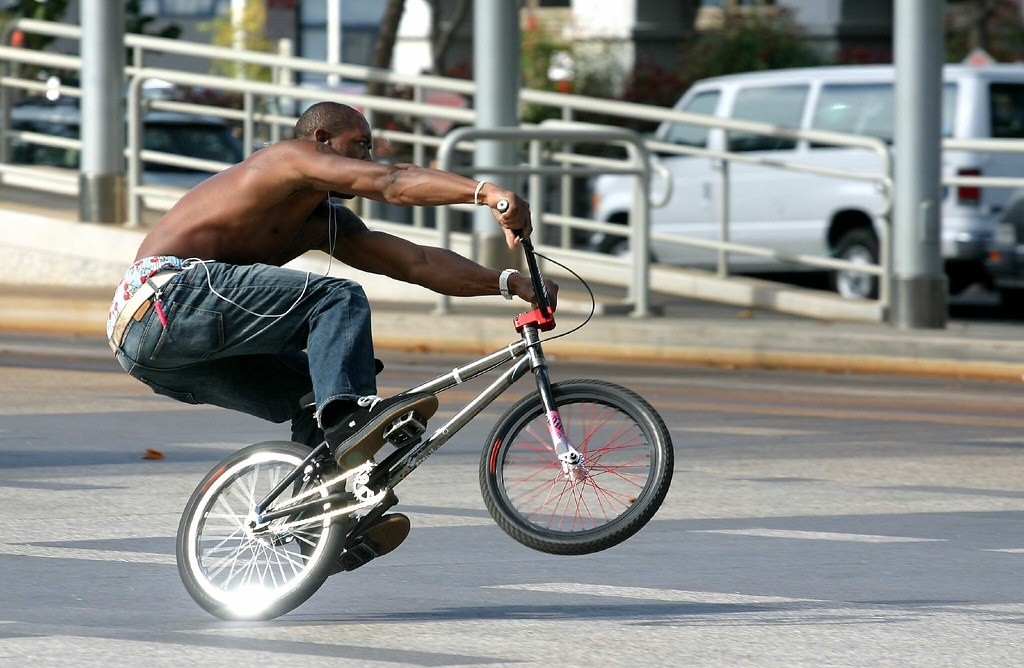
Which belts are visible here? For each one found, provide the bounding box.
[107,270,179,357]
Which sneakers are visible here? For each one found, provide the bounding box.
[300,513,410,576]
[324,391,438,470]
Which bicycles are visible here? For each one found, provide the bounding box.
[172,197,676,626]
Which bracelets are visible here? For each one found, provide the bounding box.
[475,181,486,206]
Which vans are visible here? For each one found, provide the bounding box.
[8,96,245,190]
[591,61,1024,315]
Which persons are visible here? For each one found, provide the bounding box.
[108,100,559,576]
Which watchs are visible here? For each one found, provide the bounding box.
[499,269,522,300]
